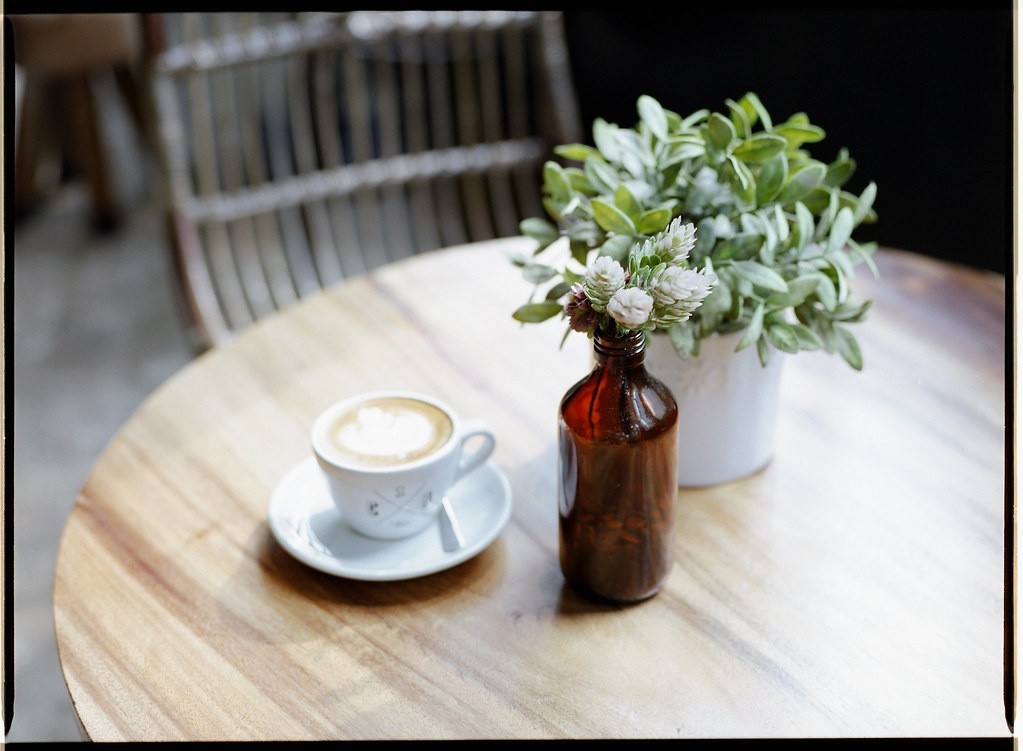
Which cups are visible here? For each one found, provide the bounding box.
[310,390,498,541]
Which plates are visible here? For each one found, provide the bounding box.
[267,458,515,582]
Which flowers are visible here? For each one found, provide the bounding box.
[564,216,715,333]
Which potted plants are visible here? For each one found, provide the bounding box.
[513,93,877,488]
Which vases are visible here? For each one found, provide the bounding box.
[556,322,677,602]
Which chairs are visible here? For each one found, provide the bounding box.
[144,12,581,349]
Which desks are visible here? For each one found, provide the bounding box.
[53,230,1005,738]
